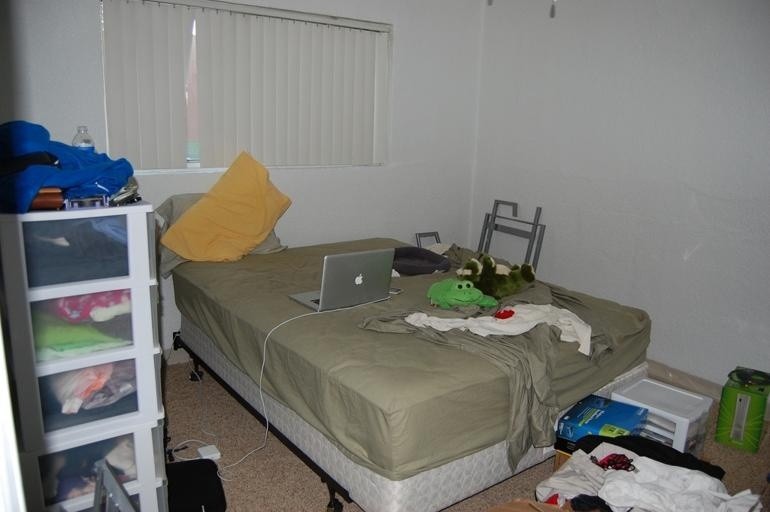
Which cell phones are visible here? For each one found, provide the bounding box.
[388,286,403,295]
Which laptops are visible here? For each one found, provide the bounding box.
[289,246,397,313]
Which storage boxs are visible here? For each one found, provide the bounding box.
[609,375,714,465]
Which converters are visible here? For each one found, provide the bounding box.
[196,443,222,462]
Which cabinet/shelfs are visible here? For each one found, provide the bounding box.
[1,197,173,512]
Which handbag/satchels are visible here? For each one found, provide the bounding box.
[390,246,450,276]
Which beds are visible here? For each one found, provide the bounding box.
[165,232,656,511]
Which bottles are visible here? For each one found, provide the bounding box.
[72,125,95,153]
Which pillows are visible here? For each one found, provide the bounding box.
[152,190,289,281]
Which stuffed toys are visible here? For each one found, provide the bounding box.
[456,252,536,298]
[427,279,499,307]
